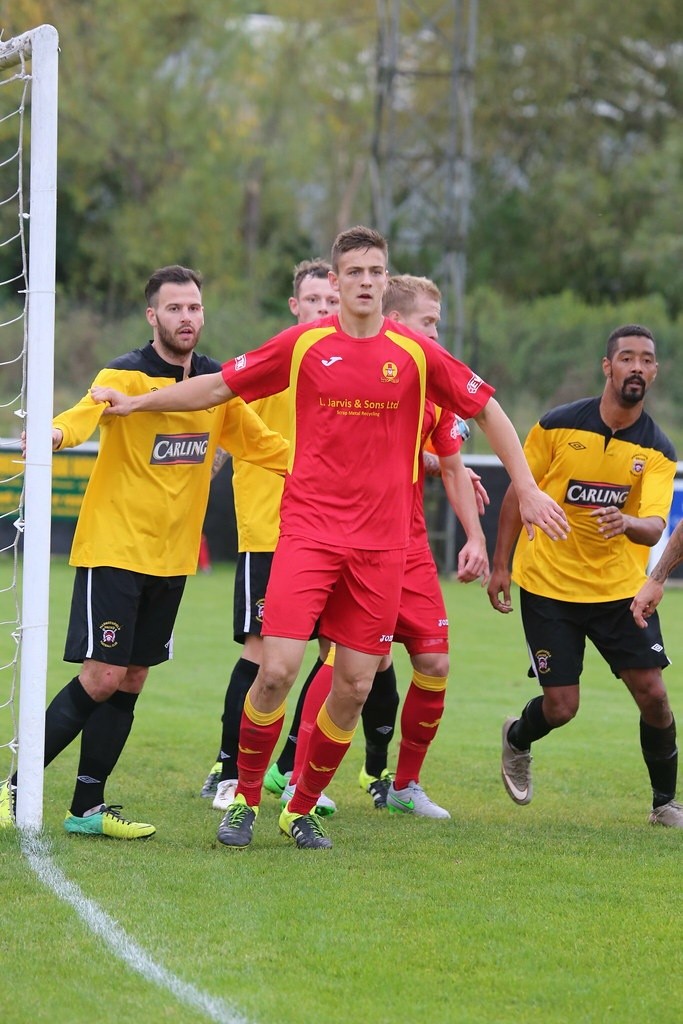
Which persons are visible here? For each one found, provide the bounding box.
[630,517,683,628]
[202,258,487,819]
[0,265,290,838]
[488,325,683,827]
[90,227,572,848]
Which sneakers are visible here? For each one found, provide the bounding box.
[277,800,335,853]
[279,780,339,816]
[213,778,240,811]
[383,779,453,822]
[359,762,395,810]
[0,781,18,828]
[216,794,258,849]
[264,763,296,797]
[500,716,536,805]
[199,762,223,798]
[63,804,158,839]
[649,800,683,830]
